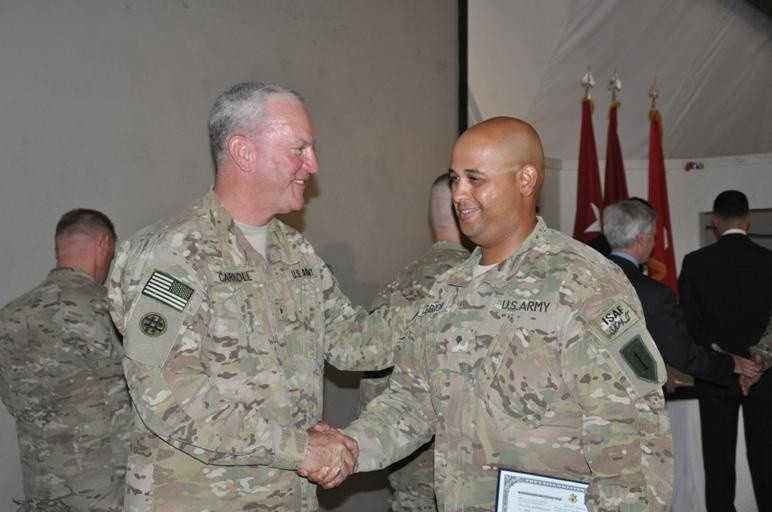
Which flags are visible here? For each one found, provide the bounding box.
[606,101,629,205]
[647,109,680,300]
[574,98,604,246]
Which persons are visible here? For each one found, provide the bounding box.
[298,116,675,512]
[602,201,759,387]
[678,190,772,511]
[585,197,655,258]
[0,208,135,512]
[359,174,476,512]
[108,81,432,512]
[740,317,772,397]
